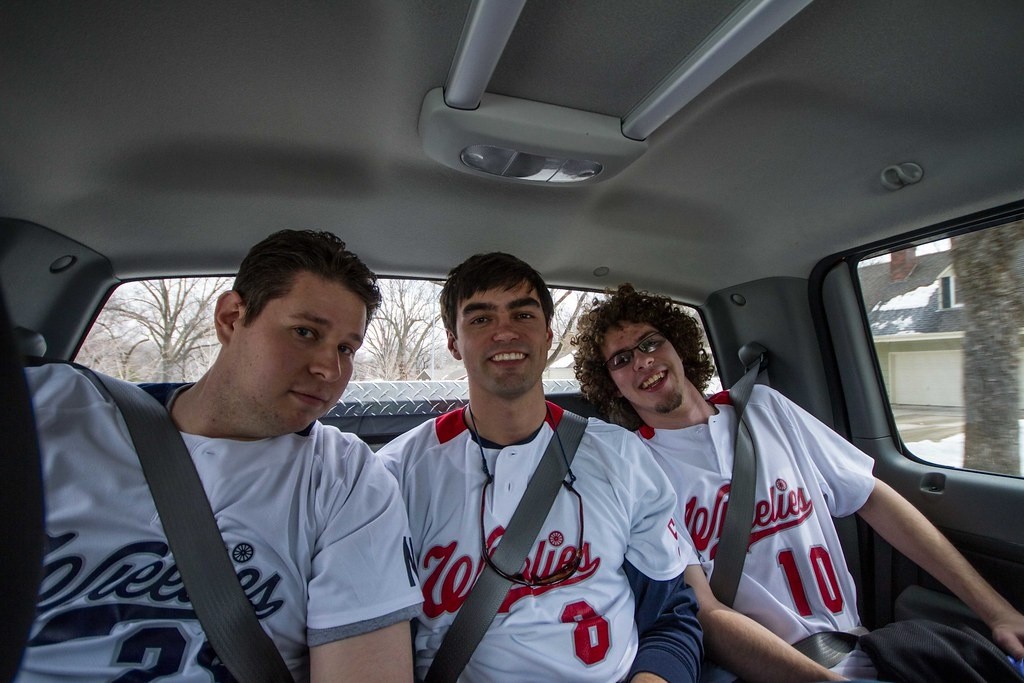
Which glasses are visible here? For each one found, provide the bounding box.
[480,474,585,587]
[601,331,668,371]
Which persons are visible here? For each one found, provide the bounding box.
[13,228,426,683]
[375,253,703,683]
[575,290,1024,683]
